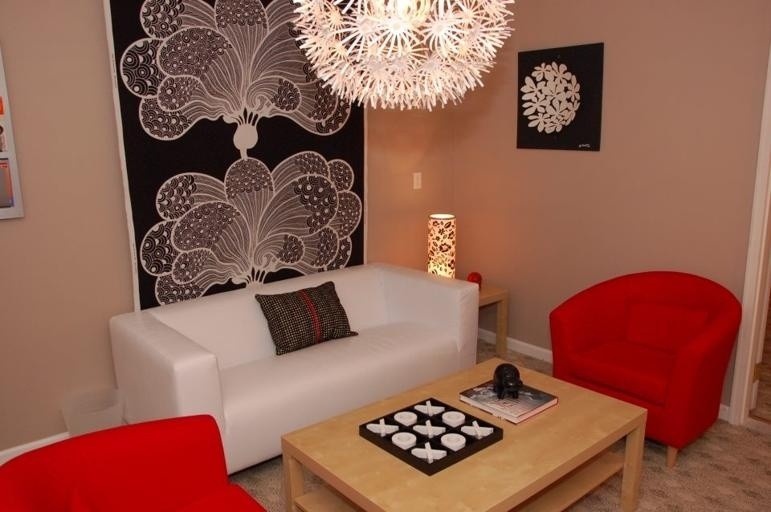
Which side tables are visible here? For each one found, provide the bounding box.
[479,283,511,356]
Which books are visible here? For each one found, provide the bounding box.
[460,378,558,424]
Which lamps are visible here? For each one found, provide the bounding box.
[291,1,518,112]
[427,213,457,280]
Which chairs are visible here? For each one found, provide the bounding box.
[548,270,744,469]
[0,414,269,512]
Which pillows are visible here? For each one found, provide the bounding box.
[254,281,359,357]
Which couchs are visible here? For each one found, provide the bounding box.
[109,261,480,477]
